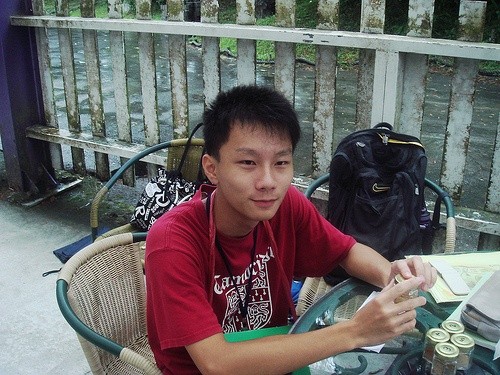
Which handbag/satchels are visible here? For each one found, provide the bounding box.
[129,122,213,233]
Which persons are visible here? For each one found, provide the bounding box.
[145,86,437,375]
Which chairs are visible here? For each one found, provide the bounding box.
[90,137,206,272]
[55,232,160,374]
[294,171,456,336]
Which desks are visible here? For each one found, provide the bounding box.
[289,248,500,375]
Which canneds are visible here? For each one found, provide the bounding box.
[391,273,419,303]
[423,320,475,375]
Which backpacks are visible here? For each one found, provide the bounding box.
[323,119,433,288]
